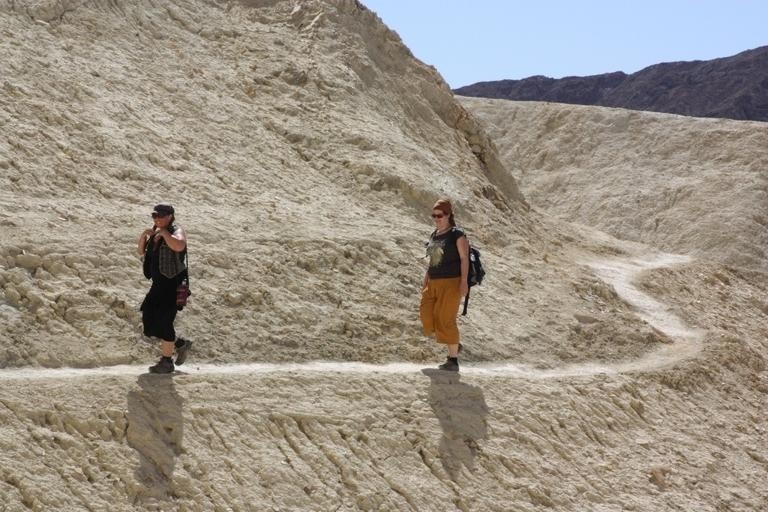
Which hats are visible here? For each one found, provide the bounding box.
[151,204,174,215]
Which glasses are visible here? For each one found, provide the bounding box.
[152,214,163,219]
[432,214,444,218]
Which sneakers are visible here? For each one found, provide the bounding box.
[439,356,458,371]
[175,340,191,366]
[149,358,174,373]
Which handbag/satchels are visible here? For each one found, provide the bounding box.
[176,284,189,306]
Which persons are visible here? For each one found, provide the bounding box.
[419,199,470,372]
[137,204,191,374]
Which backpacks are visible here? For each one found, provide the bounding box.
[469,248,485,286]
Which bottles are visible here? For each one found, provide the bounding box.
[470,249,476,273]
